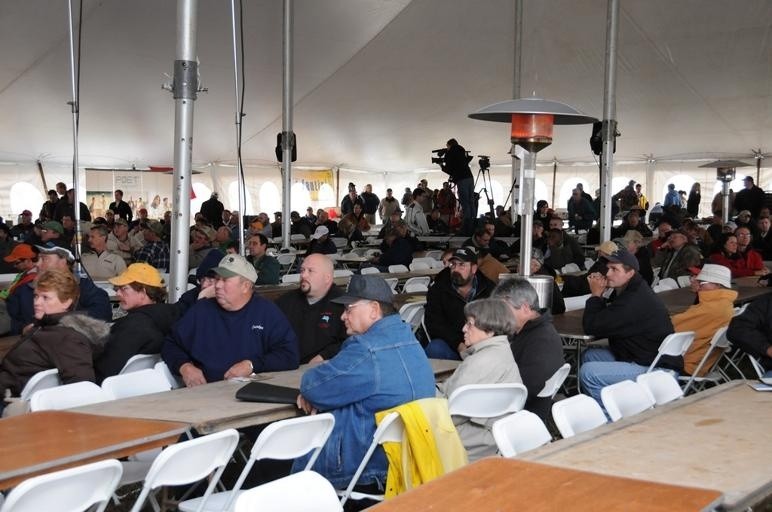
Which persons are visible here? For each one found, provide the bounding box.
[0,178,436,490]
[423,139,771,461]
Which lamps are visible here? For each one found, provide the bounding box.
[698,160,754,233]
[468,98,599,310]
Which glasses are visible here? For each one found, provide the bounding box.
[343,303,364,314]
[449,262,470,270]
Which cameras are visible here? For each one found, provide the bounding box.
[477,156,490,169]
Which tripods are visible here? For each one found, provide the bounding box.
[474,168,494,203]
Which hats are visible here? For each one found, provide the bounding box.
[689,263,732,289]
[251,222,263,230]
[105,208,163,234]
[313,226,329,239]
[109,263,167,288]
[595,230,641,272]
[723,221,736,229]
[533,220,543,226]
[742,176,754,182]
[4,208,75,263]
[195,249,258,283]
[329,275,393,305]
[448,248,478,264]
